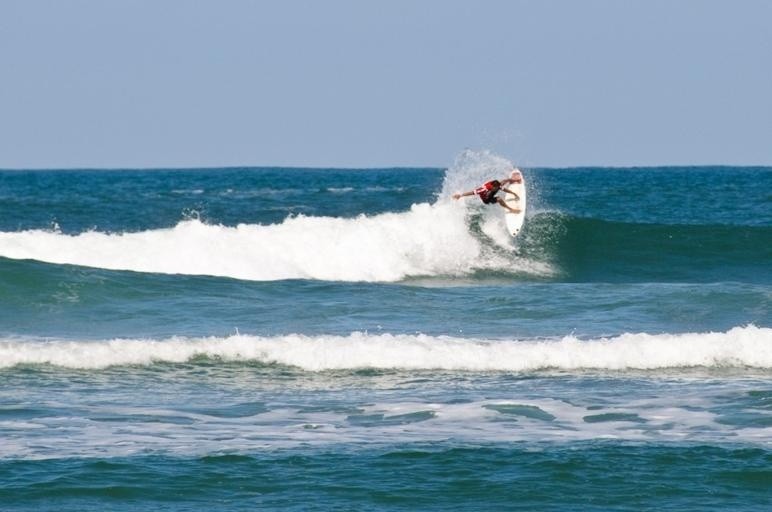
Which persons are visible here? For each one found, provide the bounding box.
[452,178,521,213]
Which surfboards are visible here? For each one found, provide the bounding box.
[505,168,526,238]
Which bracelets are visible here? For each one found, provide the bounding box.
[460,193,463,197]
